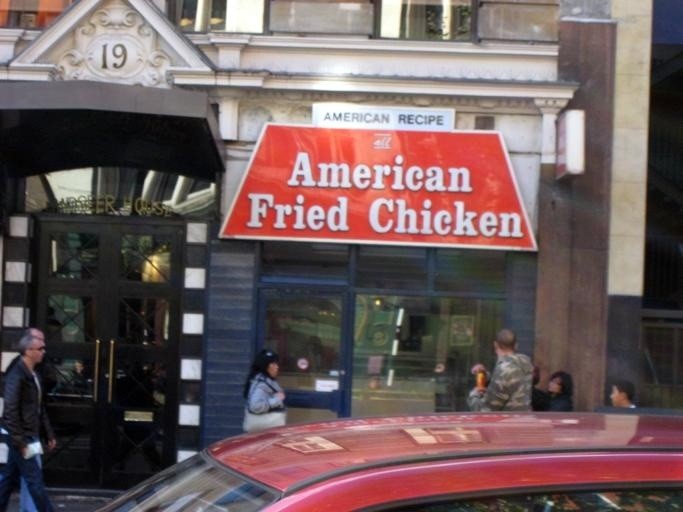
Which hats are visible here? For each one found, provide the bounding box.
[102,413,682,511]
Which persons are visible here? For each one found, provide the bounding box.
[242,350,289,434]
[467,328,535,412]
[0,327,46,511]
[0,336,58,511]
[531,371,574,411]
[605,380,636,409]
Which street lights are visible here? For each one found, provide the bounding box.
[243,404,287,433]
